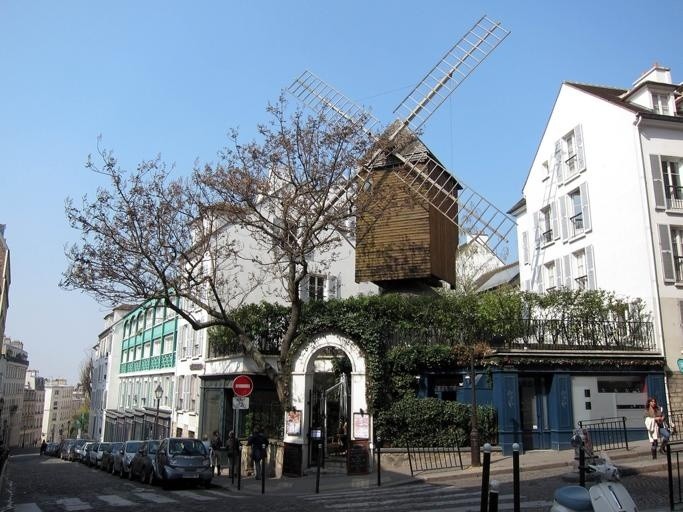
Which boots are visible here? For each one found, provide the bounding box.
[211,464,222,478]
[660,439,668,455]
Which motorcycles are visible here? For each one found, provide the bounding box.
[550,434,639,512]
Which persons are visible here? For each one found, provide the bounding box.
[200,434,209,454]
[208,430,222,476]
[223,429,242,479]
[642,396,671,461]
[245,425,268,480]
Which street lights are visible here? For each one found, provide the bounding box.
[153,384,164,440]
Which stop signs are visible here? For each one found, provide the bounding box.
[232,375,253,397]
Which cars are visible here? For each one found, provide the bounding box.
[46,439,211,489]
